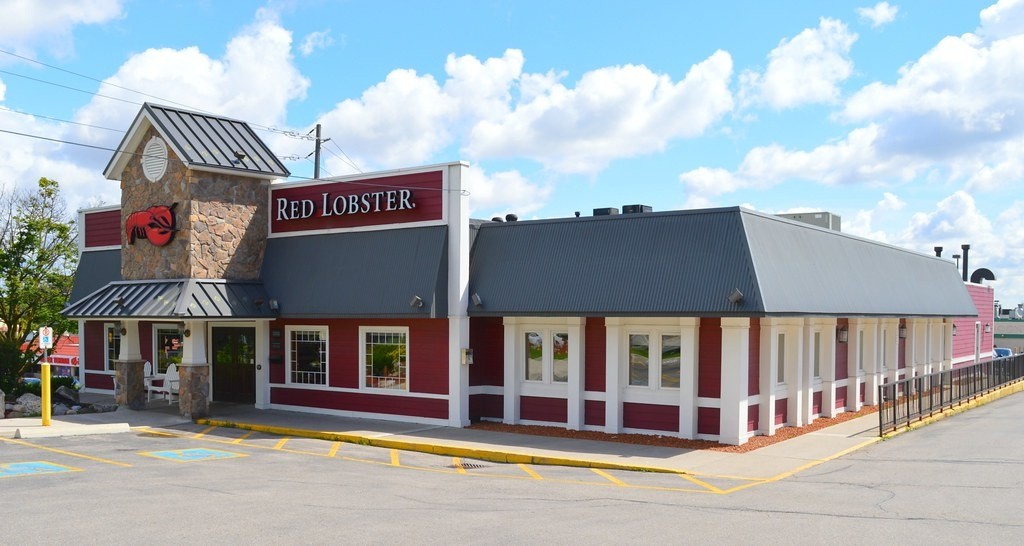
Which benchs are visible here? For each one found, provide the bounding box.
[111,362,155,400]
[147,363,185,405]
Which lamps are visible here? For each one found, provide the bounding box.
[900,324,908,338]
[728,287,744,303]
[177,321,190,338]
[983,323,990,333]
[409,295,422,309]
[836,325,848,343]
[472,293,482,306]
[114,321,127,336]
[269,298,279,311]
[953,324,957,336]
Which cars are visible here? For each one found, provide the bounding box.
[994,347,1011,359]
